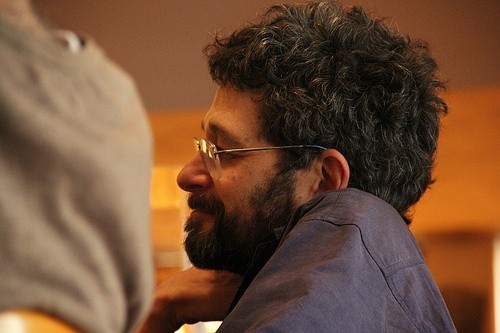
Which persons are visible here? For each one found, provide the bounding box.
[1,0,159,333]
[135,0,462,333]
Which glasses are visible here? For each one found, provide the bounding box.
[192,136,328,181]
